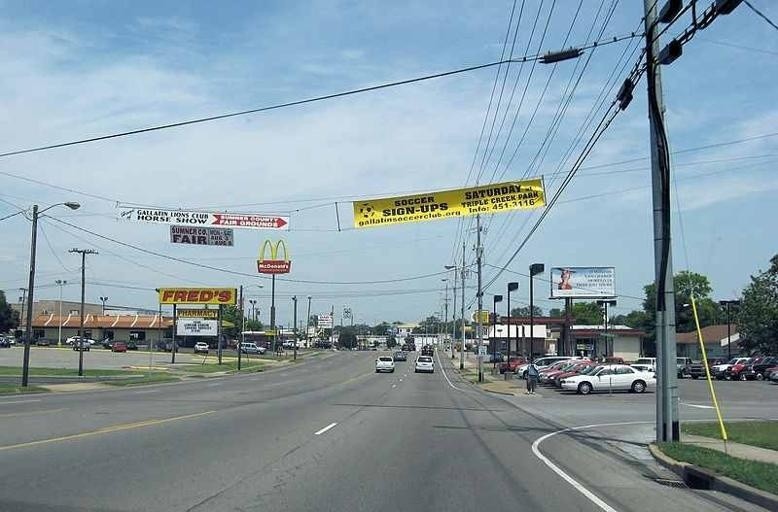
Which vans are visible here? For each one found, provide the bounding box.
[414,355,435,374]
[374,355,395,373]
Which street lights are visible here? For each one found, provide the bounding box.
[98,295,109,314]
[503,280,519,380]
[248,298,257,323]
[594,299,618,358]
[718,298,740,362]
[490,293,504,378]
[54,278,68,346]
[433,262,467,370]
[528,263,545,364]
[307,294,312,320]
[235,282,267,372]
[289,294,299,360]
[154,288,161,342]
[66,246,100,378]
[19,197,81,388]
[19,288,28,331]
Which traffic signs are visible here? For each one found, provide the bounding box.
[130,207,291,233]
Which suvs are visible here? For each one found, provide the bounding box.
[235,342,267,355]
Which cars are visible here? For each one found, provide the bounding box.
[0,333,180,353]
[557,363,656,395]
[368,342,435,362]
[192,342,210,354]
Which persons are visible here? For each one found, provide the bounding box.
[558,272,572,290]
[522,365,538,395]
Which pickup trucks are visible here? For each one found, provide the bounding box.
[281,339,301,350]
